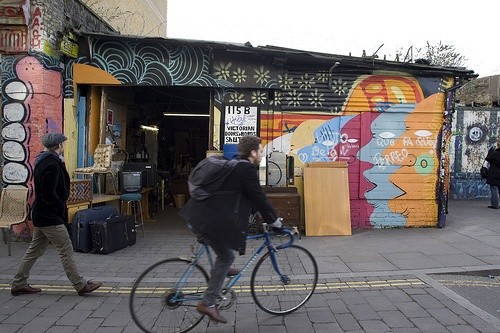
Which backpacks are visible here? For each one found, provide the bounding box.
[187,154,249,201]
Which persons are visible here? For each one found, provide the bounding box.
[181,136,283,324]
[485,138,500,209]
[10,133,102,296]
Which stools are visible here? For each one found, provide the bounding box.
[120,194,145,237]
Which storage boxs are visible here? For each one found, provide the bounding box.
[67,177,93,203]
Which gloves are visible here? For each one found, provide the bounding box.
[270,217,284,228]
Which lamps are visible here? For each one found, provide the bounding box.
[141,125,159,132]
[164,112,211,117]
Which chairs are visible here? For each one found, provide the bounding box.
[0,186,32,256]
[75,144,118,196]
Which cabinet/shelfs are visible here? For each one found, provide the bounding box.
[262,188,302,233]
[65,202,91,223]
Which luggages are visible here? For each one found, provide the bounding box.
[71,206,117,252]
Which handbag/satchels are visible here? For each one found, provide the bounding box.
[480,167,487,178]
[89,213,136,255]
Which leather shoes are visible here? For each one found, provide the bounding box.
[11,284,41,295]
[78,280,102,296]
[228,268,240,275]
[196,302,228,323]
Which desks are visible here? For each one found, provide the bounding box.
[90,188,154,224]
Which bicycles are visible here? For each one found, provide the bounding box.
[129,218,318,333]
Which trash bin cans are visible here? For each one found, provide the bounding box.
[174,194,185,208]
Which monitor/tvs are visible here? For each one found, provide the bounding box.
[117,171,142,193]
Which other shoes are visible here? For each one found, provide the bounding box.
[488,206,499,209]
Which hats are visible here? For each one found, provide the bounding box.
[42,132,68,148]
